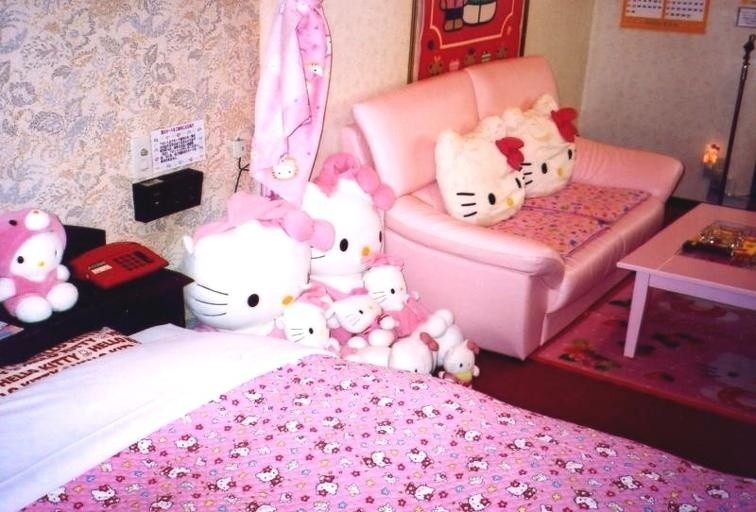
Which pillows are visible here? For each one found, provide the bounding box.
[463,55,560,120]
[352,69,479,197]
[502,94,579,198]
[434,116,524,227]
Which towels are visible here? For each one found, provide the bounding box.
[250,0,332,208]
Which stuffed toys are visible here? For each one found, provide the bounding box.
[0,207,79,324]
[180,153,482,391]
[181,153,480,390]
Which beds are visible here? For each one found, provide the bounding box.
[0,320,756,512]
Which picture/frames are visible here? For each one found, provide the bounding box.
[407,0,530,84]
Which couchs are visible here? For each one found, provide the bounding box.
[341,121,686,363]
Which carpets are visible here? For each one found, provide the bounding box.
[526,273,756,426]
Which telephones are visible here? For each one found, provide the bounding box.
[67,241,170,290]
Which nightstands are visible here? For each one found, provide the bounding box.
[0,225,195,366]
